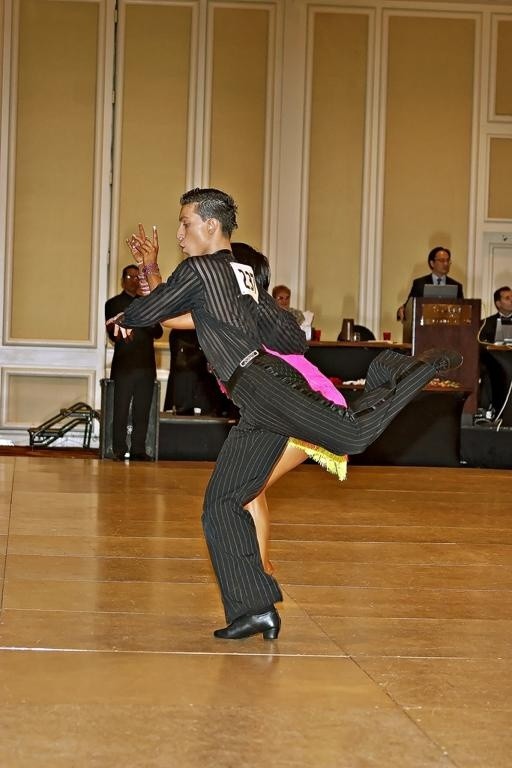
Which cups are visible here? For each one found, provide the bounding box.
[383,332,392,341]
[312,329,321,341]
[350,332,360,342]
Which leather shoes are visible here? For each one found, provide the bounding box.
[214,608,281,641]
[415,347,464,375]
[128,453,154,462]
[113,454,125,462]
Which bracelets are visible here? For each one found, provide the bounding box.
[136,260,160,296]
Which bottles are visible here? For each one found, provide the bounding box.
[343,319,354,342]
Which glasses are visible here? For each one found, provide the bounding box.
[277,295,289,300]
[434,258,451,263]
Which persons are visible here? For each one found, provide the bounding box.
[271,282,304,326]
[394,246,464,323]
[480,286,512,342]
[125,218,348,573]
[104,265,155,462]
[103,187,440,642]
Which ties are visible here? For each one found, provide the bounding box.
[436,277,442,285]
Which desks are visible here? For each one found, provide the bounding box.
[304,339,410,380]
[331,382,476,468]
[480,345,510,427]
[157,411,239,460]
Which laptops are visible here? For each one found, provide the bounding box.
[423,284,458,298]
[494,318,512,342]
